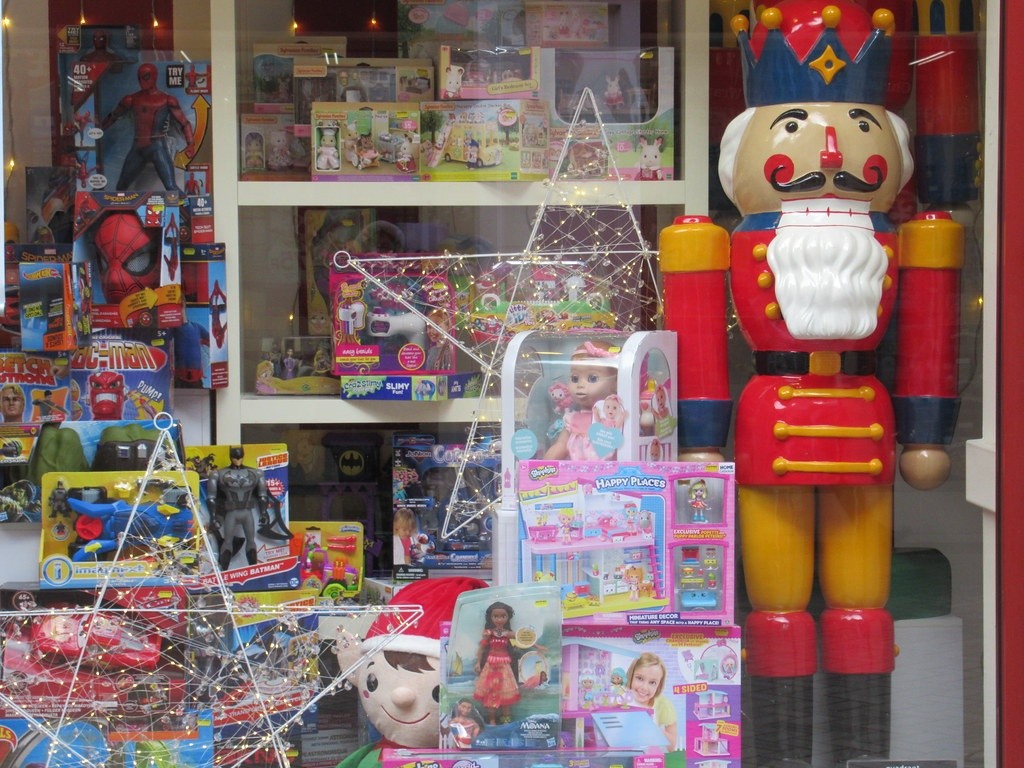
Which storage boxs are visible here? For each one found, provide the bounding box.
[290,55,435,138]
[439,621,742,768]
[0,242,228,390]
[515,459,735,627]
[214,712,302,768]
[390,429,501,570]
[252,35,347,114]
[341,372,481,402]
[71,191,184,330]
[188,586,319,727]
[0,581,188,718]
[310,0,675,182]
[185,440,301,594]
[179,194,214,244]
[69,327,174,421]
[0,708,214,768]
[19,259,92,352]
[503,329,678,512]
[38,470,200,590]
[239,101,312,181]
[382,745,666,768]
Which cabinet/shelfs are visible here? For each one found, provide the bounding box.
[207,0,709,445]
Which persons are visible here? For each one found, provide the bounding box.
[31,390,67,416]
[393,508,420,565]
[207,445,270,571]
[81,31,119,61]
[626,652,677,753]
[48,480,72,519]
[688,480,712,522]
[658,0,962,768]
[311,349,332,377]
[474,602,551,726]
[334,578,490,768]
[544,342,621,461]
[94,215,159,303]
[94,63,194,199]
[283,348,297,381]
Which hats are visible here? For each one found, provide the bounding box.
[361,577,490,659]
